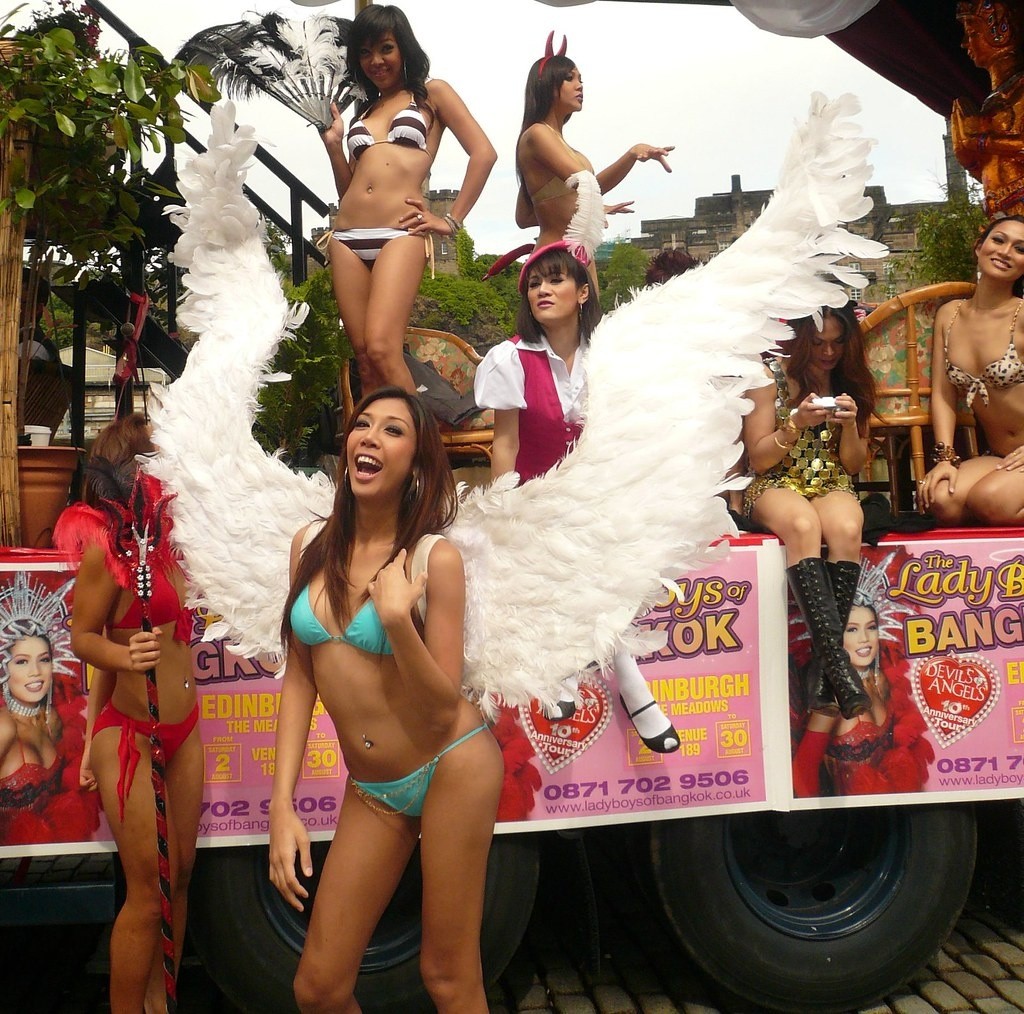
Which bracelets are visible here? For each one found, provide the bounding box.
[931,442,961,468]
[444,212,462,234]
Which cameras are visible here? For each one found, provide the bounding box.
[814,396,846,414]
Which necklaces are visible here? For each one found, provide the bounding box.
[2,683,41,717]
[856,669,870,679]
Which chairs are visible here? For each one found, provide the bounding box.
[860,282,976,518]
[335,328,495,464]
[15,360,76,445]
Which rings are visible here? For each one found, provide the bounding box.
[417,214,423,222]
[918,479,925,484]
[1012,452,1017,456]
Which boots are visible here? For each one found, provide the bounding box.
[805,561,860,711]
[786,556,872,720]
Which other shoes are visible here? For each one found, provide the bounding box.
[619,692,681,754]
[543,700,575,722]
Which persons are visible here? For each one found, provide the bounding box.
[790,601,935,799]
[68,415,203,1014]
[317,3,499,397]
[265,389,505,1014]
[645,250,744,532]
[740,300,876,719]
[916,214,1024,525]
[0,620,105,847]
[17,266,61,364]
[948,0,1024,219]
[514,31,676,303]
[473,240,683,753]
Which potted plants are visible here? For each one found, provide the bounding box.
[0,5,218,549]
